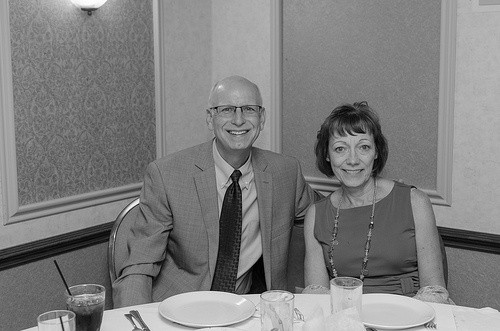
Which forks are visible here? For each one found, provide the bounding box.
[424,321,437,328]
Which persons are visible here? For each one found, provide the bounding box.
[111,76,329,309]
[297,99,446,303]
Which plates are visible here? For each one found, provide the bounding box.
[158,291,256,327]
[362,293,435,329]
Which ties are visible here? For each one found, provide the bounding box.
[209,170,242,292]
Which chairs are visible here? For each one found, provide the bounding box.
[107,194,141,284]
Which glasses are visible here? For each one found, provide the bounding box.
[209,104,262,117]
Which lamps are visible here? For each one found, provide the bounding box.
[70,0,107,16]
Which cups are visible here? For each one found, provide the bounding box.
[64,284,106,331]
[329,277,363,321]
[37,310,76,331]
[260,289,295,331]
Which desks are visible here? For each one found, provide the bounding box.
[24,293,500,331]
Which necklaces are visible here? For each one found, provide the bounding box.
[329,176,378,283]
